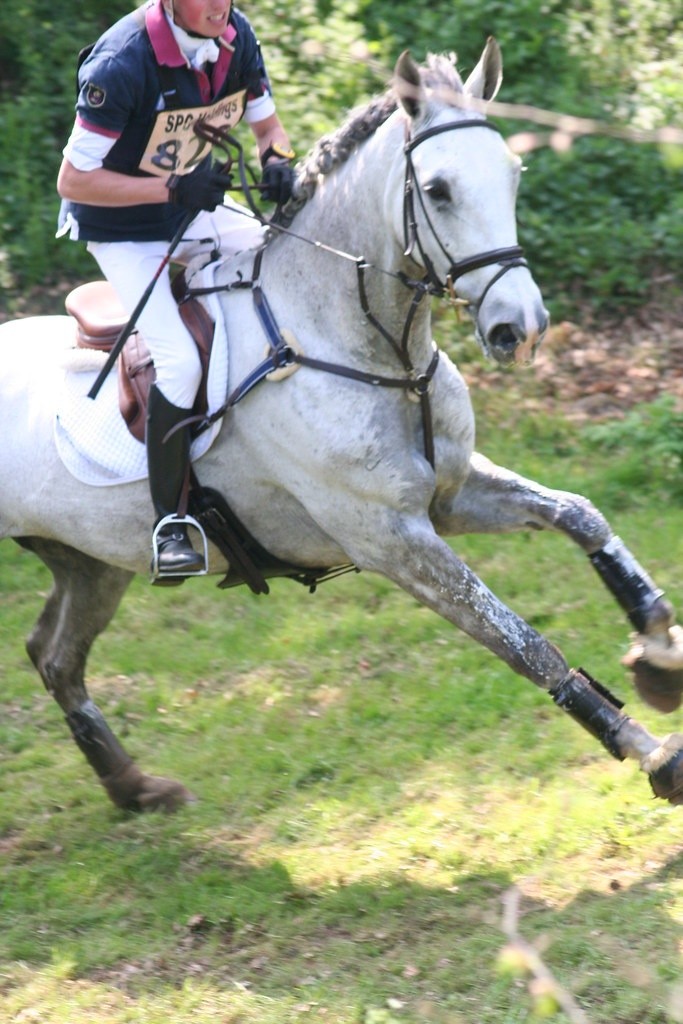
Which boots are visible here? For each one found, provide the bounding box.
[146,376,206,587]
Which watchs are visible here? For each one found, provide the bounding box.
[261,142,297,166]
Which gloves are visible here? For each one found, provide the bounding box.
[166,169,232,212]
[259,140,295,203]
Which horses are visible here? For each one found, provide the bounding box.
[0,34,683,816]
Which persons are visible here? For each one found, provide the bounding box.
[57,1,296,588]
